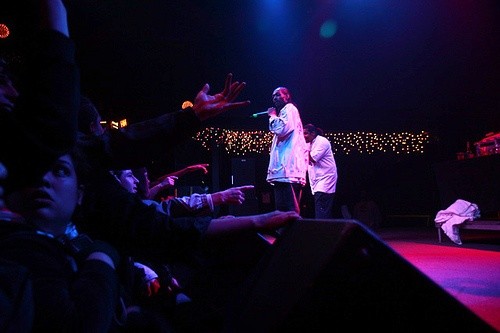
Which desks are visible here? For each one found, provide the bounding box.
[440,153,500,244]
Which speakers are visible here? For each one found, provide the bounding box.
[269,219,500,333]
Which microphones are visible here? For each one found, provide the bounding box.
[250,111,268,118]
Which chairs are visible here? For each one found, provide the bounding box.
[95,168,182,260]
[0,212,116,333]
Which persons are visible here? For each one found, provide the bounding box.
[303,124,338,219]
[265,87,309,214]
[0,0,303,333]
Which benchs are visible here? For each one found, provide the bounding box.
[435,220,500,245]
[382,214,431,228]
